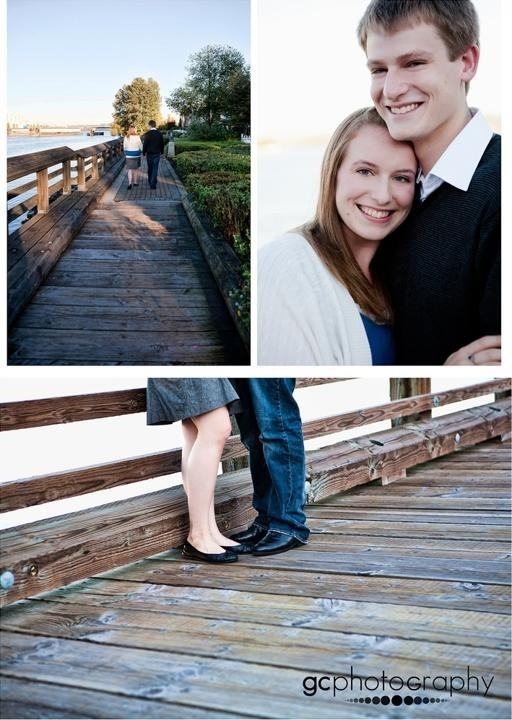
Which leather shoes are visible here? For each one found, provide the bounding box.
[181,525,304,563]
[127,184,138,190]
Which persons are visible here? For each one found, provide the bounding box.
[229,378,311,557]
[145,378,256,563]
[354,0,501,366]
[256,106,501,366]
[143,120,164,189]
[123,126,143,190]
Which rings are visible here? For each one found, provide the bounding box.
[468,353,481,365]
